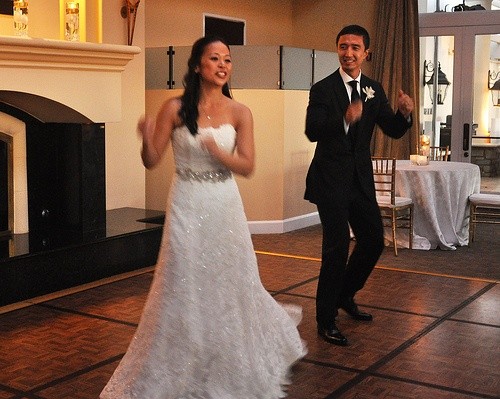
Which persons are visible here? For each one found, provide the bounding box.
[98,36,309,399]
[303,25,414,346]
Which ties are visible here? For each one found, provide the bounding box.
[348,81,360,103]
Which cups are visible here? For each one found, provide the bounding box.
[409,154,428,167]
[13,0,29,39]
[65,2,79,41]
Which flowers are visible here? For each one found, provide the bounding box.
[362,85,374,101]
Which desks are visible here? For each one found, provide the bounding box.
[371,160,481,250]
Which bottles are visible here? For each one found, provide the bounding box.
[486,131,492,143]
[419,129,430,165]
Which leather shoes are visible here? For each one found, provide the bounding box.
[317,324,348,346]
[337,296,373,321]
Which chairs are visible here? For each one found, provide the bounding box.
[429,145,449,160]
[468,192,500,247]
[371,156,414,256]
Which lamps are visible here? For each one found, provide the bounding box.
[451,2,486,11]
[424,59,449,105]
[488,70,500,106]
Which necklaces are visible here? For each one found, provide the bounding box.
[199,99,226,120]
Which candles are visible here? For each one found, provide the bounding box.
[65,1,80,43]
[12,0,28,37]
